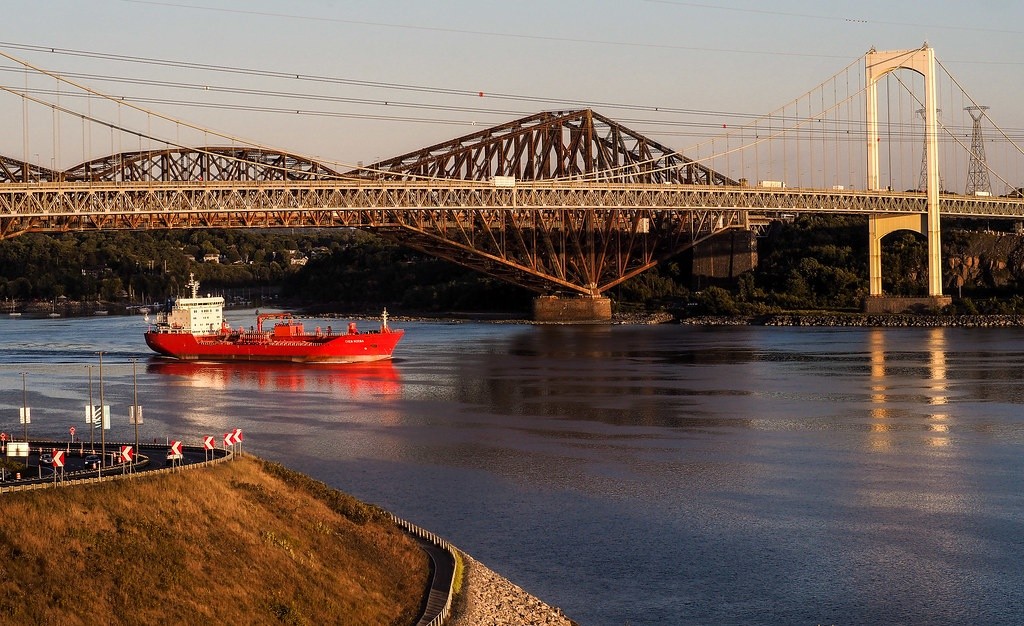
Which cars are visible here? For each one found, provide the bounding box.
[83,455,101,466]
[38,454,52,464]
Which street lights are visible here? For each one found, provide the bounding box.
[84,363,95,452]
[19,371,30,469]
[93,350,109,468]
[128,357,142,461]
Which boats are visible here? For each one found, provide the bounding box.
[143,271,405,364]
